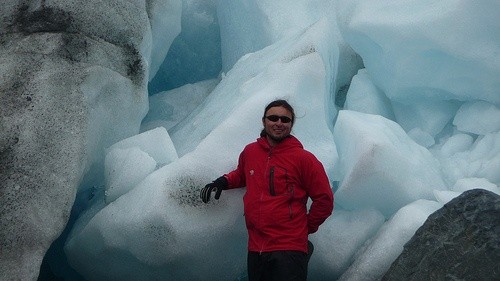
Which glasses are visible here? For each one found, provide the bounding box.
[263,115,293,124]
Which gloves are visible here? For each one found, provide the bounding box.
[200,176,227,203]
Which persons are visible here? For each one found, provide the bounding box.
[200,100,334,281]
